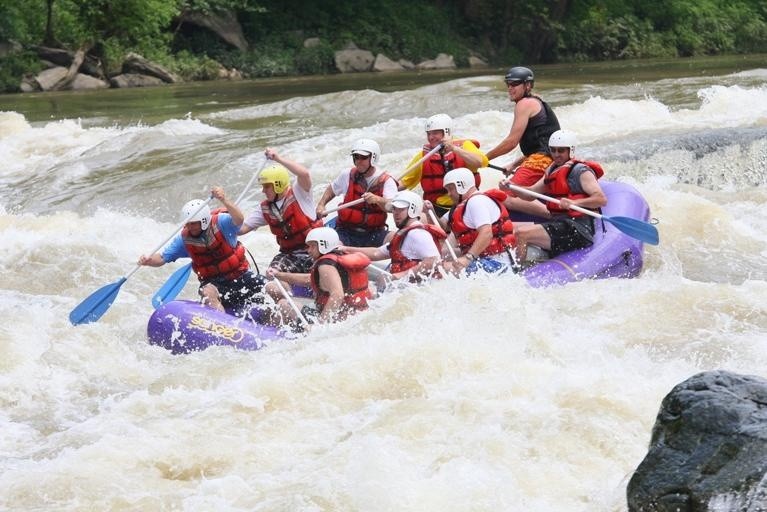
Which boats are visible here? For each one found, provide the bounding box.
[146,179,657,354]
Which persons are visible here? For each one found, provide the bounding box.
[138,186,289,315]
[398,114,488,224]
[236,146,323,298]
[422,167,517,280]
[316,139,399,247]
[275,227,371,337]
[499,130,607,271]
[482,67,560,220]
[333,191,448,295]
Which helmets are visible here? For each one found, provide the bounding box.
[505,67,534,81]
[305,227,340,255]
[392,192,422,218]
[352,140,380,167]
[183,200,211,230]
[442,168,475,194]
[548,130,576,159]
[259,166,290,192]
[425,114,453,138]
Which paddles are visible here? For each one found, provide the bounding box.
[506,182,660,246]
[151,158,270,311]
[69,195,214,326]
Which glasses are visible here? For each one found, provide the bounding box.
[507,80,523,88]
[353,155,368,160]
[551,148,566,153]
